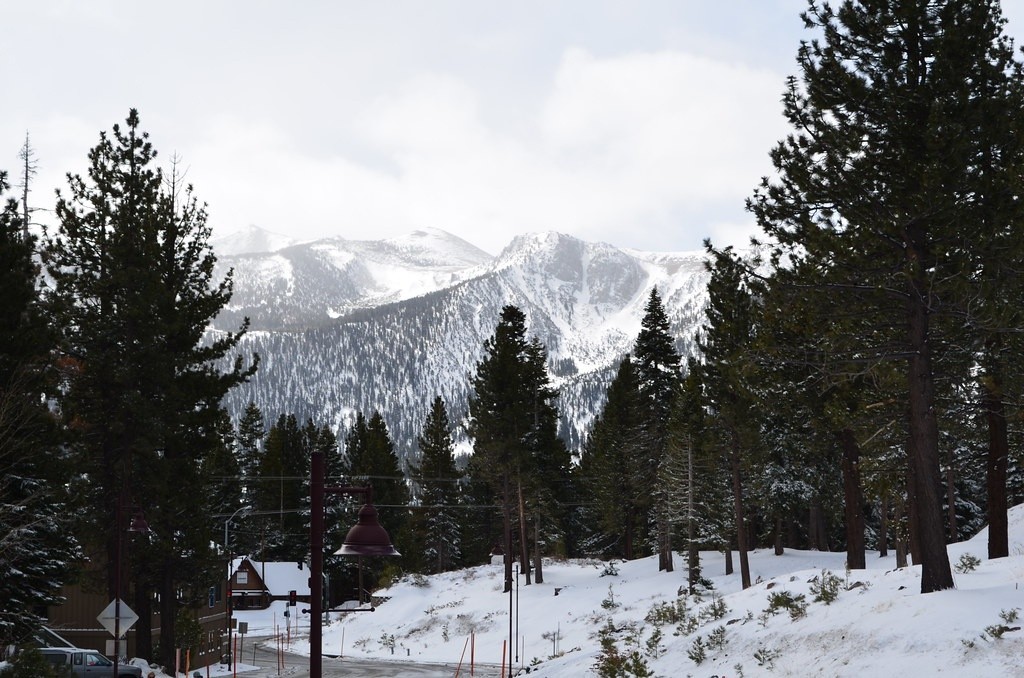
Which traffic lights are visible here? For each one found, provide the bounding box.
[298,559,302,570]
[290,590,296,606]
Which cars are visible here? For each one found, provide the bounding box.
[0,648,143,678]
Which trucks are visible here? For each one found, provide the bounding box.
[343,588,370,600]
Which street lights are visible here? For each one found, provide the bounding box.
[114,501,152,678]
[224,506,252,548]
[310,451,401,678]
